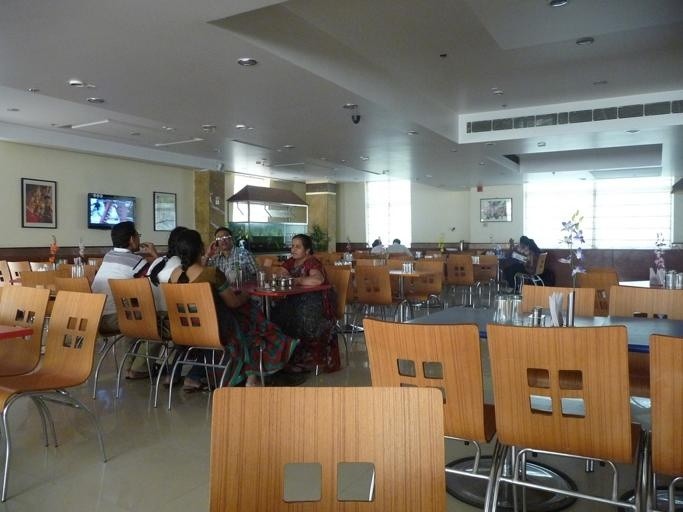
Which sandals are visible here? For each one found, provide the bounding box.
[244,380,274,387]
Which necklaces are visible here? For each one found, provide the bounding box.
[293,261,303,273]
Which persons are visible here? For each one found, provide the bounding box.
[379,239,412,257]
[501,238,539,295]
[370,238,385,258]
[205,231,258,282]
[87,221,166,379]
[498,236,529,293]
[167,232,300,387]
[146,225,216,394]
[267,235,346,375]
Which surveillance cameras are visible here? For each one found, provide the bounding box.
[352,115,360,124]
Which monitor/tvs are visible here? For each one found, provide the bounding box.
[88,193,136,230]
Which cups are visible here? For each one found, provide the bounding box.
[257,271,297,291]
[493,294,523,323]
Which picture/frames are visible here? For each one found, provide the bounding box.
[20,177,57,229]
[153,191,178,233]
[480,198,512,223]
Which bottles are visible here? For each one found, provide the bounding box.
[71,257,85,278]
[532,304,542,316]
[334,251,353,265]
[401,262,415,274]
[460,240,464,252]
[665,266,683,289]
[224,262,248,290]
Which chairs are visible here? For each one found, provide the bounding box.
[0,247,683,509]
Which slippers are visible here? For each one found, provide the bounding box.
[163,376,183,389]
[124,372,155,381]
[182,381,215,394]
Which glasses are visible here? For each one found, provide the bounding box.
[134,233,142,237]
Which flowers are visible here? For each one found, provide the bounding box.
[558,209,586,288]
[654,232,665,272]
